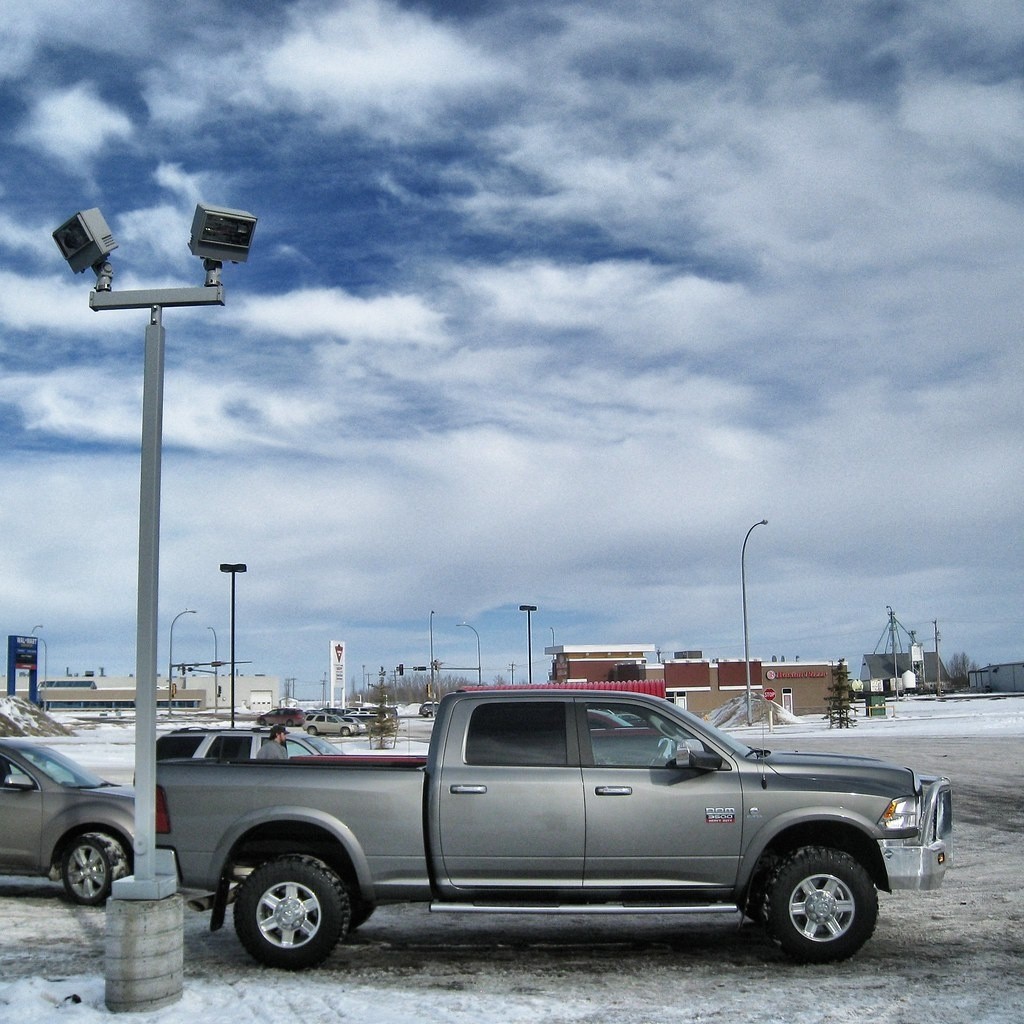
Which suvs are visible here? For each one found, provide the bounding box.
[418,701,440,718]
[257,708,306,729]
[155,724,344,760]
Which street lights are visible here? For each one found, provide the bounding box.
[455,623,483,686]
[519,603,538,684]
[741,519,772,727]
[886,605,899,702]
[362,665,365,698]
[828,659,834,697]
[51,205,259,905]
[219,563,248,728]
[38,637,47,713]
[168,610,197,717]
[207,626,218,717]
[429,610,435,717]
[549,626,556,678]
[364,674,373,695]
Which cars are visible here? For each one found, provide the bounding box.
[0,737,136,908]
[586,706,672,738]
[304,702,399,737]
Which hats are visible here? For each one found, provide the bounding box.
[270,726,291,735]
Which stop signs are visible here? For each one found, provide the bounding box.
[764,688,775,701]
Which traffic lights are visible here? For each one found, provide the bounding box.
[172,683,177,695]
[217,685,222,698]
[182,666,185,675]
[425,684,431,698]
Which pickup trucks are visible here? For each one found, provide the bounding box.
[151,687,957,972]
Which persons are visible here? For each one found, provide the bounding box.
[256,725,292,760]
